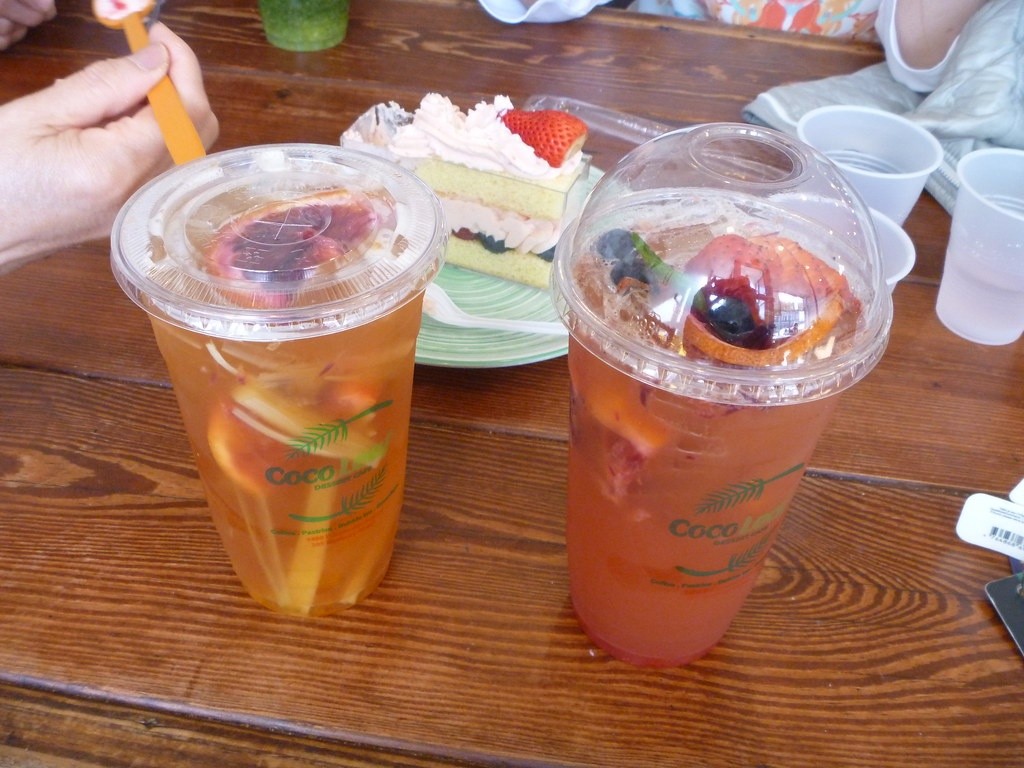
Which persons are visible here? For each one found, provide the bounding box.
[0,0,220,281]
[468,1,1024,155]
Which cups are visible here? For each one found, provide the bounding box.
[933,147,1024,346]
[791,104,945,231]
[109,143,453,623]
[542,120,897,673]
[762,193,914,301]
[258,0,355,53]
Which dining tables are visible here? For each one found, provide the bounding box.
[1,0,1024,768]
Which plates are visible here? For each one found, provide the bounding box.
[409,159,640,379]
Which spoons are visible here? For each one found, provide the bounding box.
[91,2,206,166]
[418,279,570,338]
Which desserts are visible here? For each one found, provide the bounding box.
[338,93,593,290]
